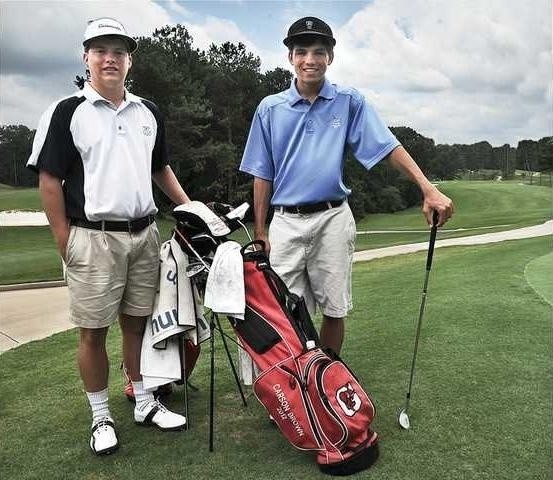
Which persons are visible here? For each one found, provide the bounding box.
[239,17,455,357]
[25,17,191,455]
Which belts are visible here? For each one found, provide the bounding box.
[274,202,341,215]
[70,215,154,234]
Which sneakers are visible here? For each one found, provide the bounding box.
[134,397,186,433]
[90,416,119,455]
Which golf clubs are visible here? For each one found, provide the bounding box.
[172,201,250,280]
[399,208,438,428]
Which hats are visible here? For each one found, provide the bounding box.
[83,17,139,53]
[283,16,336,45]
